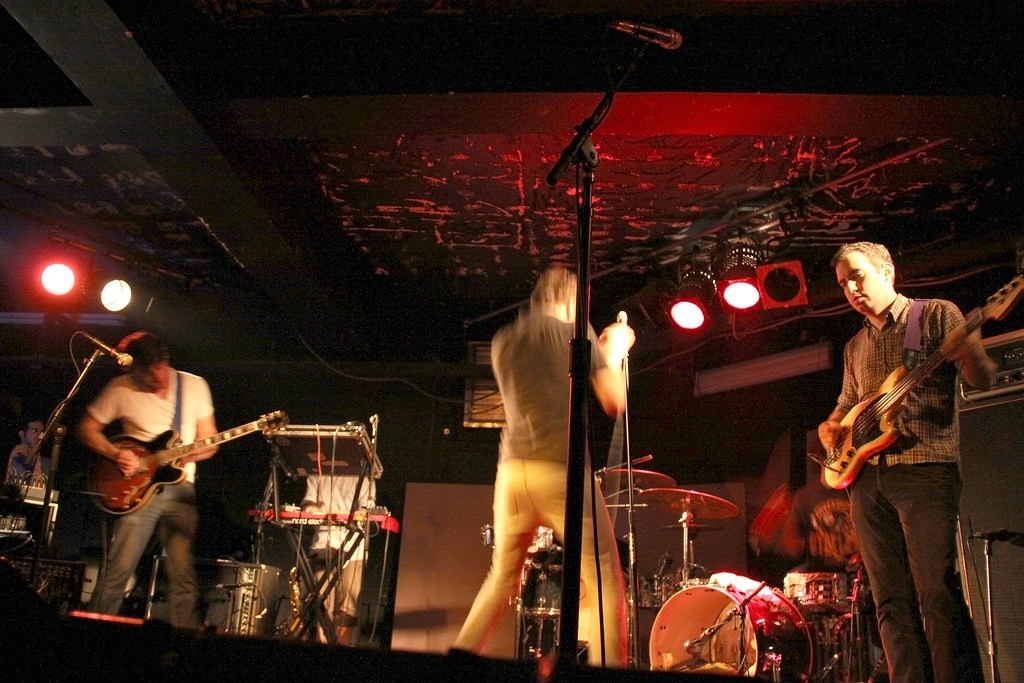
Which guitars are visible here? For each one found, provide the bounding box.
[821,275,1024,490]
[85,410,289,516]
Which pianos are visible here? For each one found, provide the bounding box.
[247,509,399,646]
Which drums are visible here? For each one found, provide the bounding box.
[783,573,849,612]
[627,576,681,608]
[648,573,814,683]
[482,525,564,618]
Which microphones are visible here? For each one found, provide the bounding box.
[659,552,673,576]
[616,311,628,324]
[81,332,133,366]
[369,414,380,424]
[971,527,1010,541]
[610,19,682,50]
[684,638,704,654]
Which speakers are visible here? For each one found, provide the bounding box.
[954,388,1024,683]
[145,555,282,637]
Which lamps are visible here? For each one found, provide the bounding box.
[670,245,718,328]
[79,267,132,312]
[722,228,764,309]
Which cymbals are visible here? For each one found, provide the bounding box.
[600,469,676,493]
[637,488,739,520]
[661,524,724,532]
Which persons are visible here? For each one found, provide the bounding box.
[75,330,218,629]
[301,475,377,647]
[455,264,636,669]
[0,416,57,555]
[817,242,996,683]
